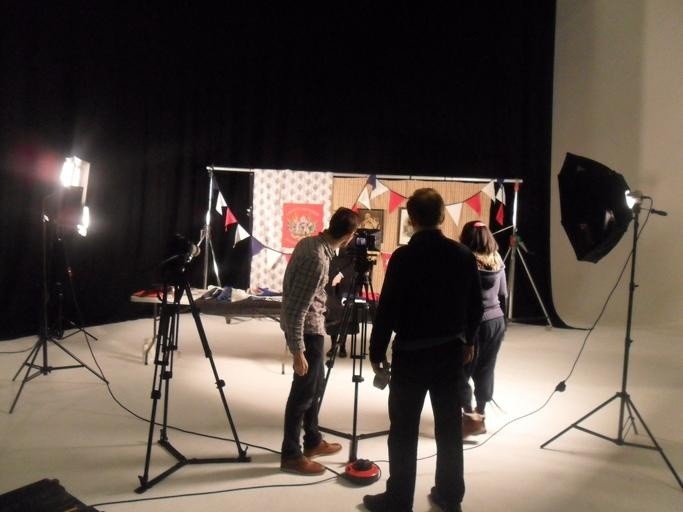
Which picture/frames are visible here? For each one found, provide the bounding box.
[396,207,415,247]
[357,208,384,243]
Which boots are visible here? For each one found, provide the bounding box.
[462,412,480,439]
[471,411,487,437]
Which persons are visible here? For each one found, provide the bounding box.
[280,207,342,475]
[323,230,363,357]
[364,187,484,512]
[458,221,509,434]
[359,212,378,229]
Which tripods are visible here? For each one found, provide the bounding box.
[47,202,97,343]
[541,208,683,489]
[134,259,251,494]
[502,182,554,329]
[300,270,392,464]
[176,171,221,290]
[9,215,109,414]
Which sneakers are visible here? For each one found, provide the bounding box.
[338,348,347,359]
[362,492,414,512]
[430,486,463,511]
[326,346,338,357]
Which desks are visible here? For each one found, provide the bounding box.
[130,281,379,377]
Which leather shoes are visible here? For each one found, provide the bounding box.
[280,455,327,476]
[303,438,343,460]
